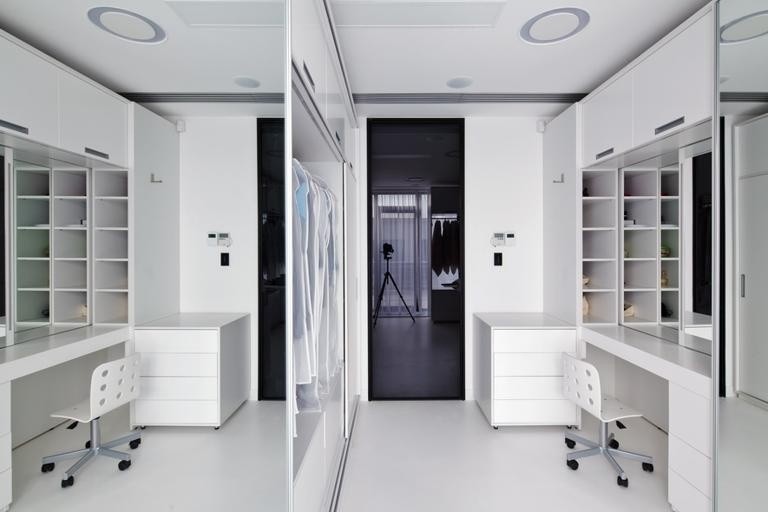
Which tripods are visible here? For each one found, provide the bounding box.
[374,260,415,328]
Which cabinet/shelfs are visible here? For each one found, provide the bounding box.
[0,27,250,511]
[472,0,768,512]
[286,0,358,512]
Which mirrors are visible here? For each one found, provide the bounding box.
[0,0,286,511]
